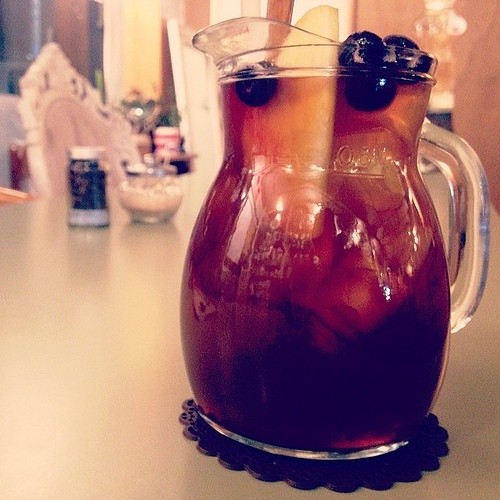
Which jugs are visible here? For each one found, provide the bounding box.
[178,17,491,470]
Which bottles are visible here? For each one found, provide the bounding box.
[67,142,113,228]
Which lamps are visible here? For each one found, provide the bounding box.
[412,1,469,41]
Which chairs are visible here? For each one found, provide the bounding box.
[17,42,130,200]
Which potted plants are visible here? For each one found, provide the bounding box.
[117,89,157,154]
[151,96,183,159]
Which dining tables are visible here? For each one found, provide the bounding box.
[0,155,500,500]
